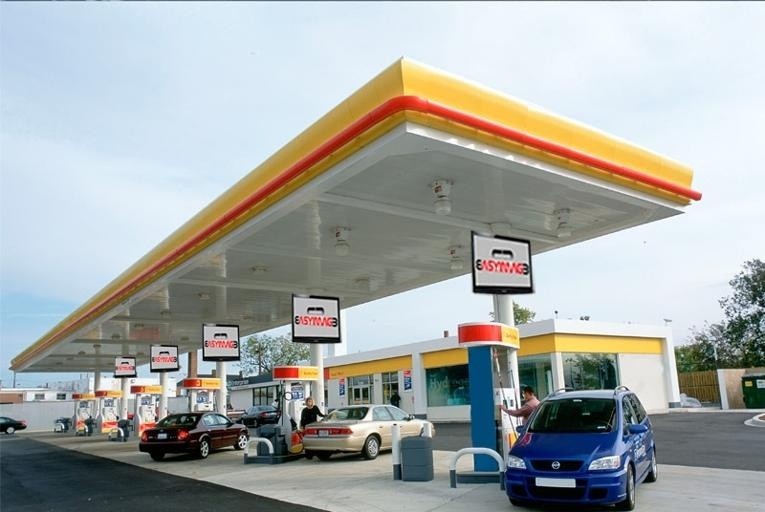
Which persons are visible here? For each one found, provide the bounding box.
[300,397,327,428]
[390,391,400,408]
[499,385,540,426]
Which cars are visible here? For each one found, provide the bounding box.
[504,386,657,511]
[139,404,435,462]
[54,418,72,432]
[682,397,702,408]
[0,416,28,434]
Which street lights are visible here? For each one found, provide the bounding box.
[709,337,719,369]
[566,358,578,389]
